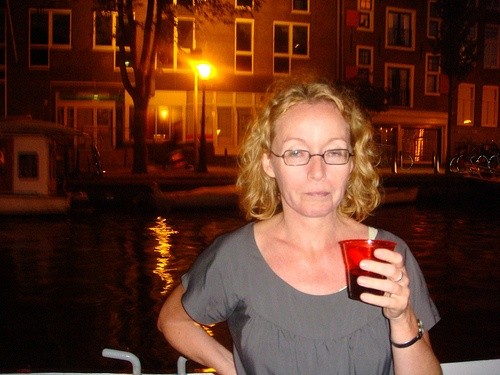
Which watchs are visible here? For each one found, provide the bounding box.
[389,319,424,349]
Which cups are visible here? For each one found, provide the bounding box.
[338,239,398,302]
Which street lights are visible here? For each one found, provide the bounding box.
[191,59,214,173]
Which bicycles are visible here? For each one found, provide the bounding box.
[447,139,500,178]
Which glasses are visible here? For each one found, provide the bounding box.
[269,148,355,167]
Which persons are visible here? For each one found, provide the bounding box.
[158,70,444,375]
